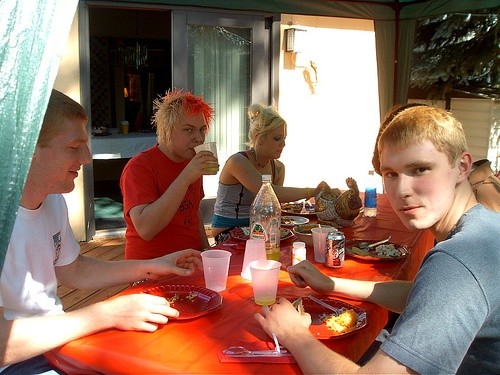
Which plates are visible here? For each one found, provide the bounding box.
[139,285,224,320]
[281,205,317,215]
[286,297,367,341]
[280,215,309,226]
[230,227,293,242]
[345,239,412,262]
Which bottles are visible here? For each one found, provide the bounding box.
[249,175,281,261]
[364,171,377,217]
[291,241,306,267]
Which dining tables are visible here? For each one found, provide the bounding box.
[43,190,434,375]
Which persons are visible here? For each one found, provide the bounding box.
[0,88,206,375]
[119,87,220,288]
[253,103,500,375]
[211,101,341,246]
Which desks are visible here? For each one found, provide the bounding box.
[92,127,159,159]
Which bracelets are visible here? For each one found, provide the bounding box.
[306,188,311,201]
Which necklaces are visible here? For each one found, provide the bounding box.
[252,149,269,168]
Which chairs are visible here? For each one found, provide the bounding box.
[200,198,216,225]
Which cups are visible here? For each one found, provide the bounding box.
[200,249,232,290]
[193,142,219,172]
[120,120,129,134]
[311,227,338,263]
[249,259,282,305]
[240,239,267,280]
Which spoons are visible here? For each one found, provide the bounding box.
[223,346,288,355]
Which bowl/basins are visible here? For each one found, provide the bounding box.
[293,224,339,246]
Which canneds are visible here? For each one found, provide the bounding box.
[325,231,345,268]
